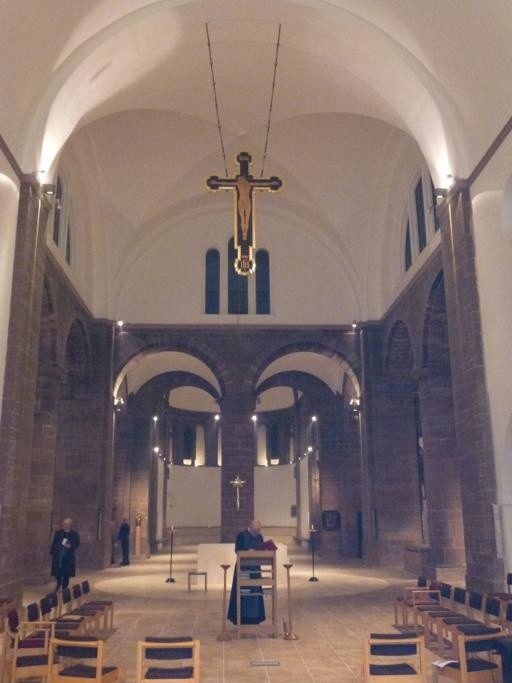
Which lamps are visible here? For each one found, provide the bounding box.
[347,393,363,413]
[113,392,126,412]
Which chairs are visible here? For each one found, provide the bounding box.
[1,578,128,682]
[390,575,511,683]
[360,624,425,683]
[132,629,204,683]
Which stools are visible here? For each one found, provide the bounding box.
[187,571,208,591]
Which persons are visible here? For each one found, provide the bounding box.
[229,520,267,623]
[115,519,131,564]
[211,175,272,241]
[48,516,79,590]
